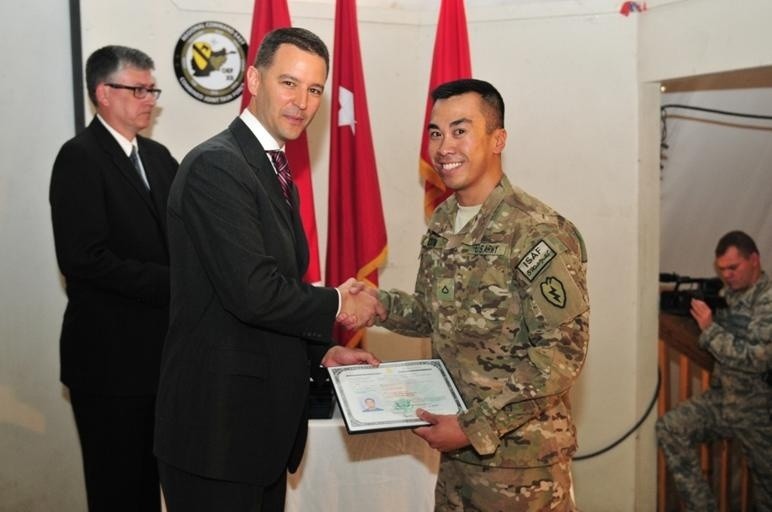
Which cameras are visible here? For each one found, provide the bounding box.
[658,277,729,318]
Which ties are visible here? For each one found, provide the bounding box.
[130,146,142,177]
[270,150,294,211]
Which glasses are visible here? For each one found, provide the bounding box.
[102,81,161,100]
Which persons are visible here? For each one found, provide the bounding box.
[655,230,772,512]
[154,28,388,512]
[336,79,589,512]
[50,45,180,512]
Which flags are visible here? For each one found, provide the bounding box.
[324,0,390,347]
[240,0,324,283]
[420,0,473,225]
